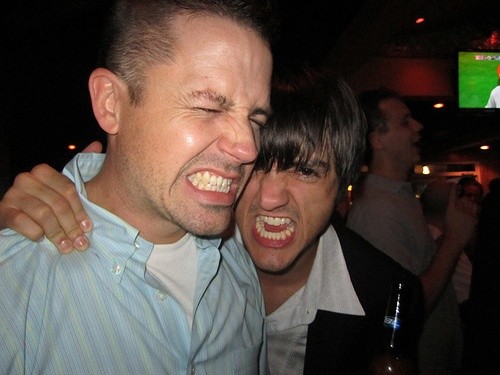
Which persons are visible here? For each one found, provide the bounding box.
[0,74,425,375]
[345,85,500,370]
[0,0,424,375]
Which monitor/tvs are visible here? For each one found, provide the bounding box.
[455,48,500,112]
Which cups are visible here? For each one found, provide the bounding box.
[454,176,483,213]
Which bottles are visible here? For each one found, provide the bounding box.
[365,281,415,375]
